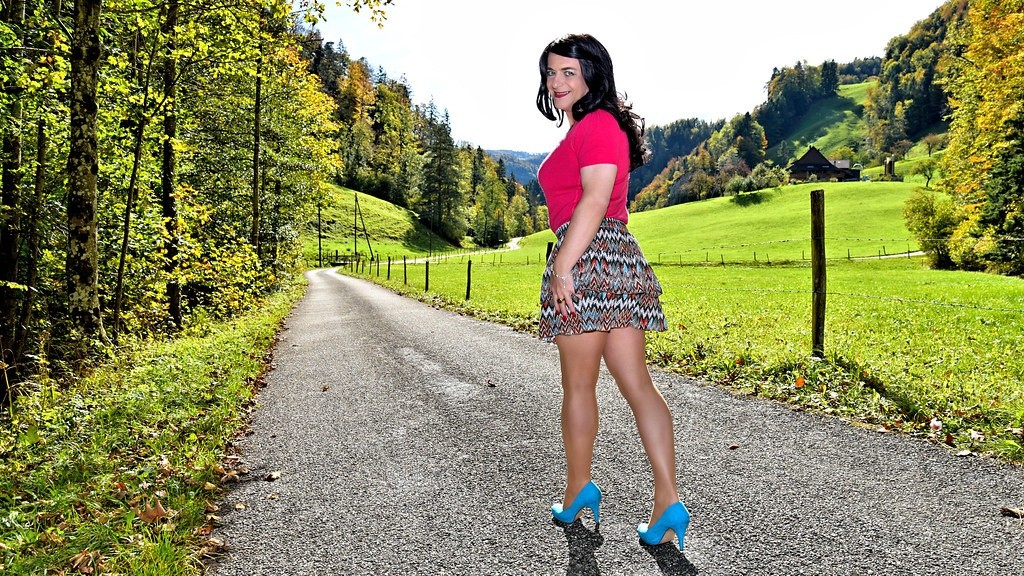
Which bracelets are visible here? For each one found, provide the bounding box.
[549,263,576,288]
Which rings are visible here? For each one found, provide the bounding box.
[557,298,565,304]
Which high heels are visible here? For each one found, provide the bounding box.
[551,481,602,524]
[638,501,690,550]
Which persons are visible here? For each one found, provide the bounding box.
[536,33,691,552]
